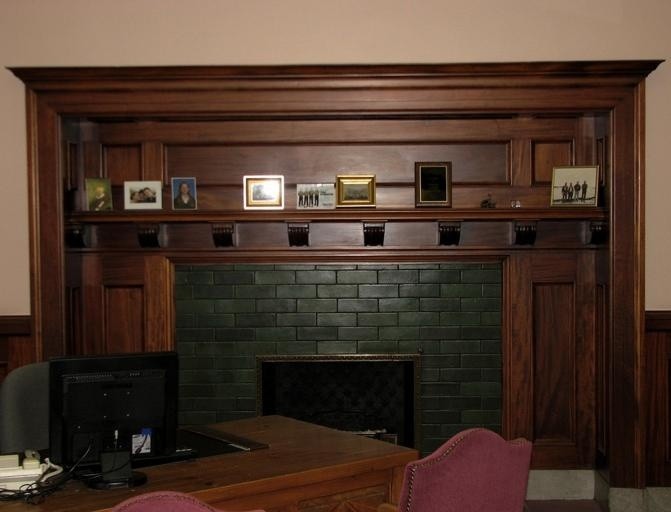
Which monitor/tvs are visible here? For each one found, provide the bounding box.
[48,349,181,490]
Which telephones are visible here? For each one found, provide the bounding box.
[0,450,42,491]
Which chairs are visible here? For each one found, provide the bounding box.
[0,361,49,455]
[379,427,534,512]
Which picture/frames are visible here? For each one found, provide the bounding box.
[549,164,601,210]
[413,160,454,209]
[84,176,198,213]
[242,174,285,211]
[335,174,377,209]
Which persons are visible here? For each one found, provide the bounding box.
[142,186,155,202]
[129,190,139,202]
[562,182,569,200]
[567,182,574,199]
[297,187,303,207]
[314,189,319,207]
[308,187,314,206]
[303,188,308,207]
[90,186,110,211]
[137,189,147,202]
[174,183,195,208]
[573,181,580,201]
[581,179,588,202]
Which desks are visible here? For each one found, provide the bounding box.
[0,411,421,512]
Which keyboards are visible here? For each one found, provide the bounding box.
[76,444,199,469]
